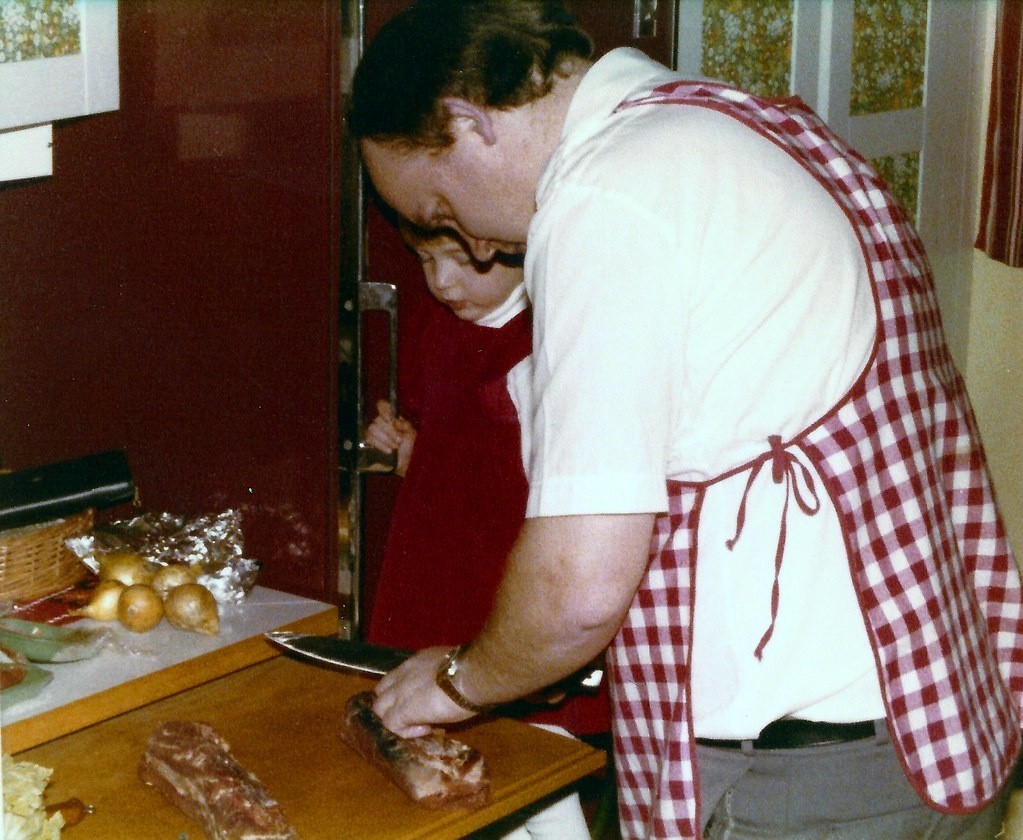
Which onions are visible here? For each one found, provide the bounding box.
[63,547,218,632]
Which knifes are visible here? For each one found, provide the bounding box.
[264,633,573,704]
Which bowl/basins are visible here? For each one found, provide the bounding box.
[0,618,109,662]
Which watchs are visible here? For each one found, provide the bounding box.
[436,641,499,713]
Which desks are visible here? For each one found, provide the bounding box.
[0,584,606,840]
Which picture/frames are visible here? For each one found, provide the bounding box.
[0,0,120,130]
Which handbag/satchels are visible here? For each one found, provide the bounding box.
[0,449,139,529]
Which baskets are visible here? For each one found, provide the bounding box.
[0,509,95,617]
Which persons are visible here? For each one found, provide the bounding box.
[351,0,1023,840]
[365,222,615,829]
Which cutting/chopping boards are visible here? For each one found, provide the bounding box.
[10,653,607,840]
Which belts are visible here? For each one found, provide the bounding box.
[693,720,875,750]
